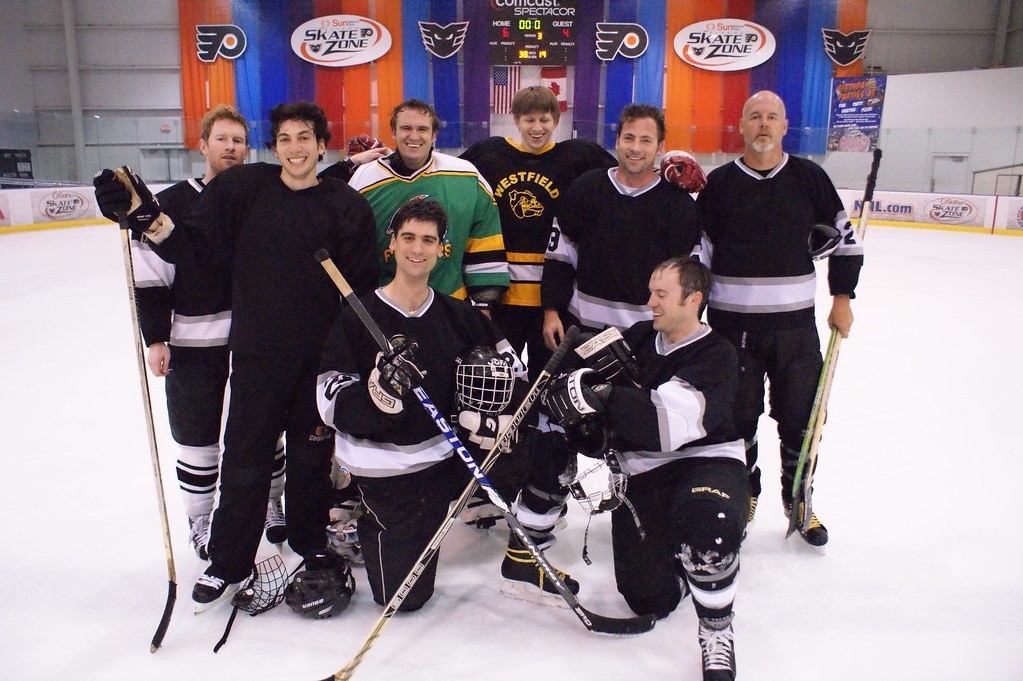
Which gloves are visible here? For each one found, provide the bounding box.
[661,150,708,193]
[541,367,612,428]
[368,334,429,415]
[94,163,174,246]
[573,326,640,389]
[346,133,385,157]
[458,410,520,453]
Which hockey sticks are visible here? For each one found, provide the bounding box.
[314,324,579,681]
[115,203,179,655]
[314,246,658,637]
[783,149,883,540]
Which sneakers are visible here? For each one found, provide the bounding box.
[191,563,240,616]
[746,496,758,530]
[781,488,829,556]
[189,513,212,564]
[550,503,568,531]
[698,612,736,681]
[501,527,580,609]
[263,496,288,555]
[463,492,511,531]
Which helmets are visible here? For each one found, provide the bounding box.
[325,523,365,565]
[452,343,515,418]
[285,549,355,620]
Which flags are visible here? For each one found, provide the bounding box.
[493,66,521,114]
[539,64,569,113]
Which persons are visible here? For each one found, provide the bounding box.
[128,106,393,569]
[315,198,581,611]
[90,100,380,616]
[344,83,708,387]
[697,89,862,556]
[541,104,712,352]
[571,257,753,681]
[347,98,510,305]
